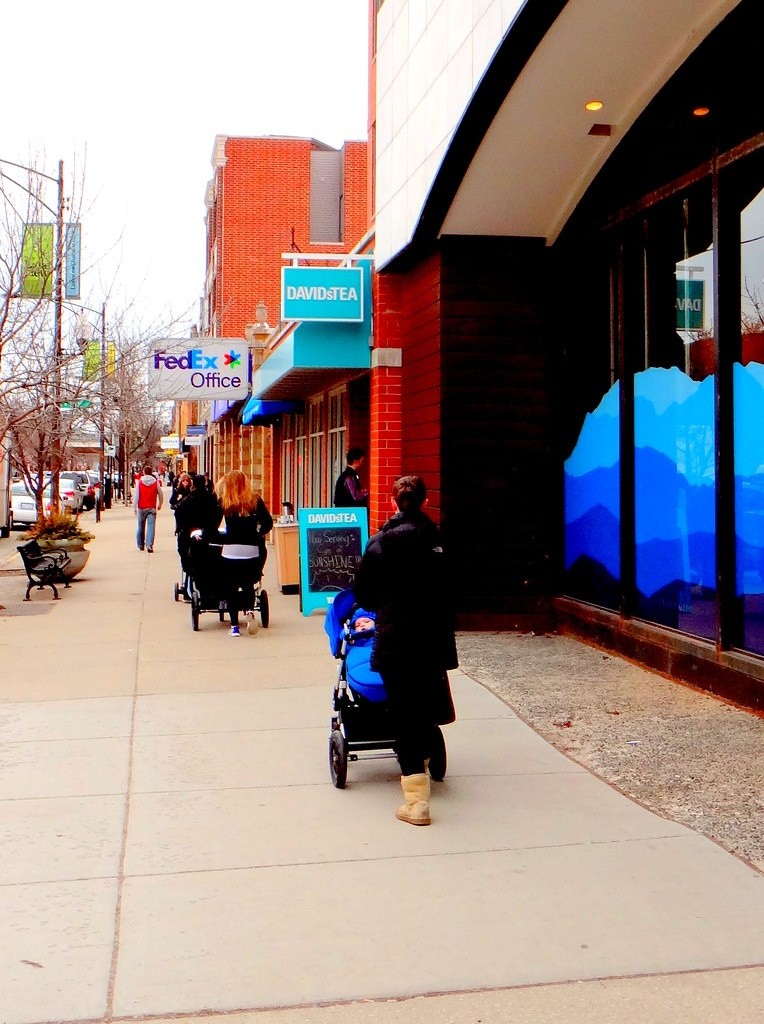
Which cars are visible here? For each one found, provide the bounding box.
[9,470,123,523]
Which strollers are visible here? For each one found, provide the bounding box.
[326,588,447,789]
[174,530,270,631]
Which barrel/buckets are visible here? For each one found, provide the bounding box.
[282,502,291,516]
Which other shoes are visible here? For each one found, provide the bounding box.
[138,547,144,550]
[147,548,153,553]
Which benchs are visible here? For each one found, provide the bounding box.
[14,538,74,601]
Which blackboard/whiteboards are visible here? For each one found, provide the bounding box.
[299,507,369,609]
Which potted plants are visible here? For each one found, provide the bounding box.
[17,498,99,580]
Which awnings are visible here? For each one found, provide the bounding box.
[251,249,372,400]
[242,395,303,427]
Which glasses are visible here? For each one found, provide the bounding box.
[181,479,189,482]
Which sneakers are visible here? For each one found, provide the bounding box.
[246,611,258,635]
[228,625,240,636]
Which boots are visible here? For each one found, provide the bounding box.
[394,758,430,824]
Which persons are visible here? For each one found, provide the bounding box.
[333,448,368,508]
[351,474,460,824]
[169,469,273,638]
[134,466,163,553]
[347,608,375,633]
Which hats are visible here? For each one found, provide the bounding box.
[203,472,209,475]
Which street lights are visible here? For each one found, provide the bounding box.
[1,292,107,511]
